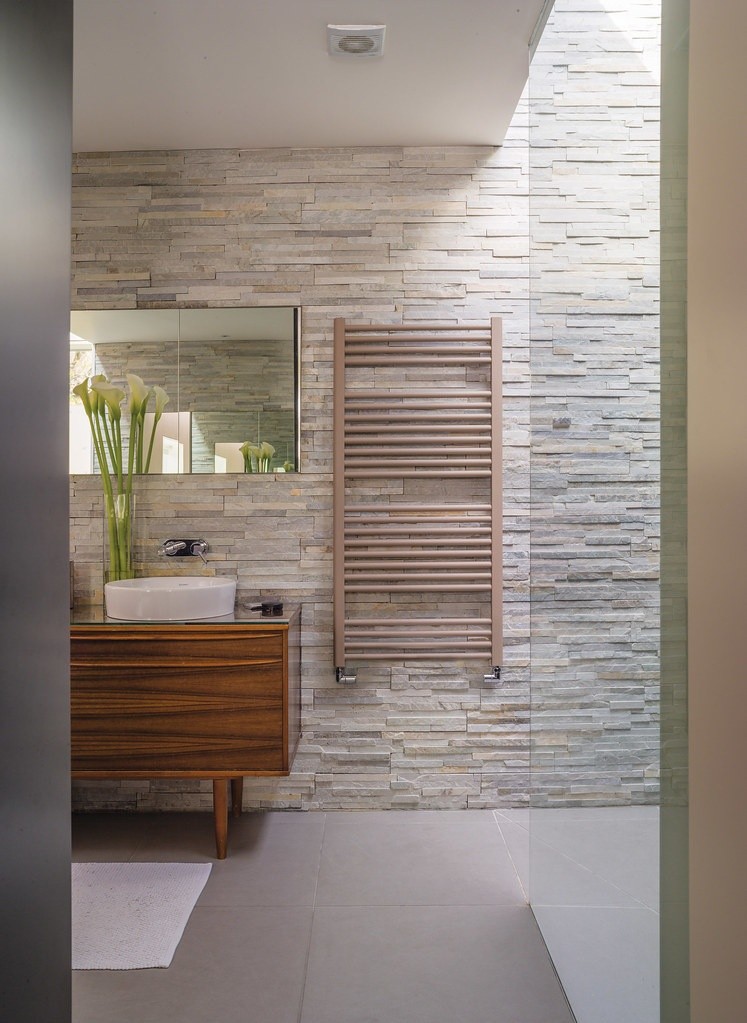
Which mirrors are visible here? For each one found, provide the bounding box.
[69,307,300,475]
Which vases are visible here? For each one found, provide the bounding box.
[102,494,136,610]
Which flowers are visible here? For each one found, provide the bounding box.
[238,439,295,473]
[72,372,170,493]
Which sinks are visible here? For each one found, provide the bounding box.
[104,576,236,621]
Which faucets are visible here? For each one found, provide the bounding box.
[156,541,186,557]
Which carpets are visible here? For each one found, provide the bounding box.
[71,861,214,970]
